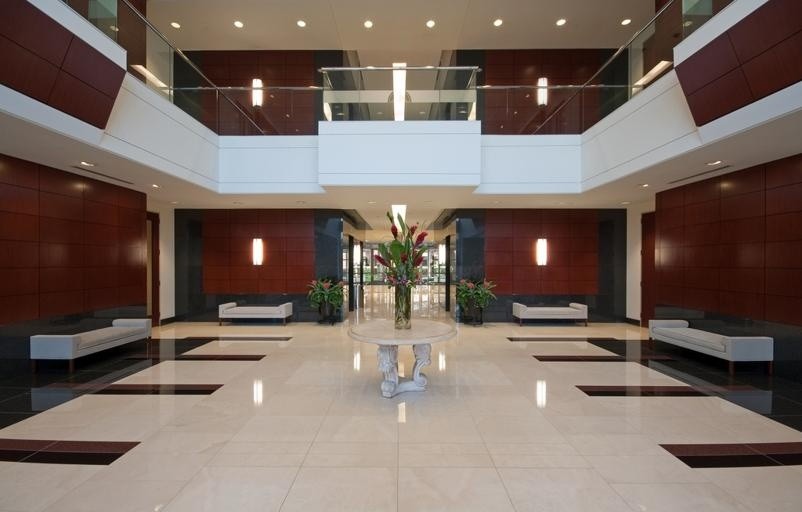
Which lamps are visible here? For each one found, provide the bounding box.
[252,237,264,267]
[536,78,548,108]
[251,78,265,109]
[536,238,548,267]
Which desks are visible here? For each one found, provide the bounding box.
[349,318,457,398]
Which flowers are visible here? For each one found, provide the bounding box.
[307,277,345,307]
[453,278,496,310]
[373,211,429,289]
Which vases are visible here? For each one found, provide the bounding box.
[463,306,482,323]
[320,300,336,317]
[395,285,412,329]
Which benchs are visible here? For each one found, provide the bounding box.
[29,317,152,375]
[512,302,588,328]
[217,301,293,325]
[649,319,774,376]
